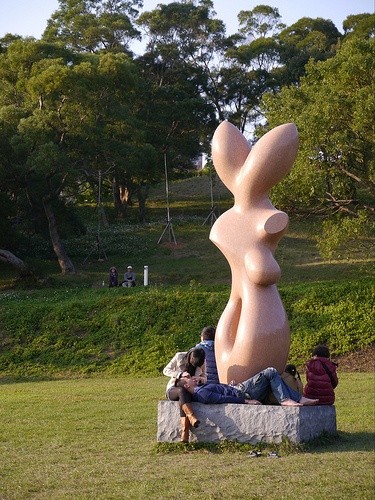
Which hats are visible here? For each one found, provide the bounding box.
[110,267,117,271]
[285,364,296,376]
[127,266,132,269]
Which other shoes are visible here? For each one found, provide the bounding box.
[248,451,262,457]
[267,451,279,458]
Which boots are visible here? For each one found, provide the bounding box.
[180,416,190,443]
[182,403,200,428]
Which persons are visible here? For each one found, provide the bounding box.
[175,367,318,406]
[122,266,135,287]
[282,364,303,395]
[187,326,219,385]
[108,267,118,286]
[163,349,207,444]
[302,346,338,406]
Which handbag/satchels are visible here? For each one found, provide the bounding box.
[331,377,337,389]
